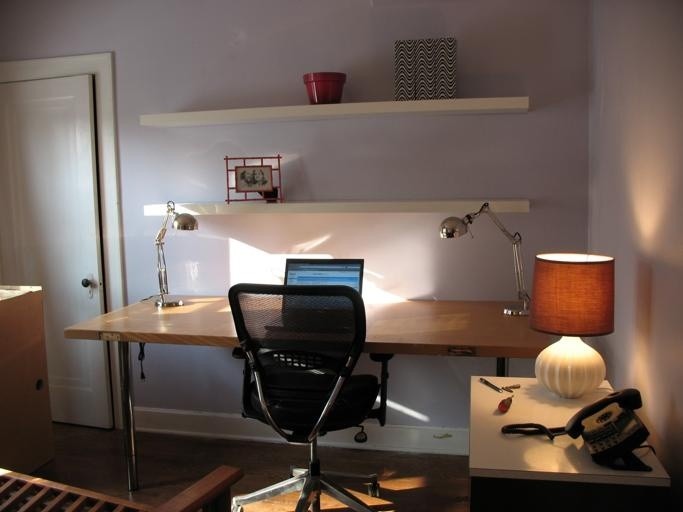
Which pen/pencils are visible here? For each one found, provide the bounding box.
[480,378,503,393]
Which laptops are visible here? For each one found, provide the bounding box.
[264,258,364,331]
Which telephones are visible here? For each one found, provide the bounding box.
[501,388,652,472]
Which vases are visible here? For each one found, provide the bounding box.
[303,72,346,103]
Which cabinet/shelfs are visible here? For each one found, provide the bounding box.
[140,97,531,215]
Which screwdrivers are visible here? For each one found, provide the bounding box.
[498,395,514,412]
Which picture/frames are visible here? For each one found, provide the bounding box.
[223,154,285,204]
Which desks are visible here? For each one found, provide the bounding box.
[65,296,608,491]
[470,375,671,512]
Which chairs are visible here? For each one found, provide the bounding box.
[228,284,394,512]
[0,465,244,512]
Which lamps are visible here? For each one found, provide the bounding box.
[439,202,531,316]
[529,253,615,399]
[154,200,198,307]
